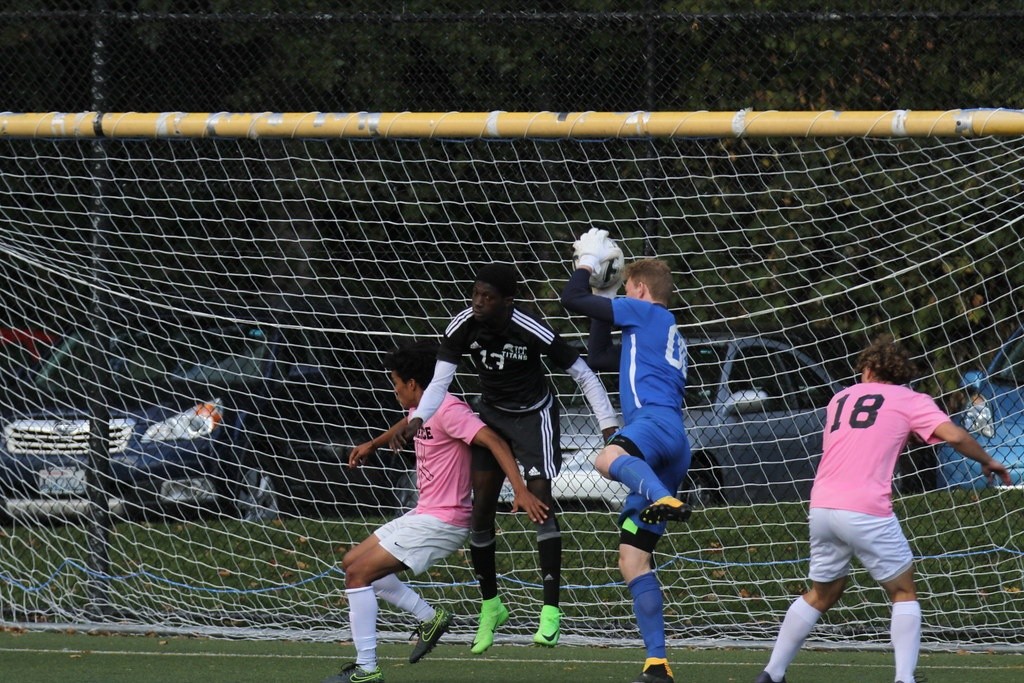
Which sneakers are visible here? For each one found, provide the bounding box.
[533,605,561,648]
[639,657,674,683]
[640,497,692,525]
[471,596,508,655]
[409,607,452,664]
[322,664,384,683]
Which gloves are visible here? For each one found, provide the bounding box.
[573,227,621,275]
[592,272,629,299]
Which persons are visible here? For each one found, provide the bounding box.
[324,344,549,683]
[562,227,691,682]
[755,340,1009,683]
[390,262,618,653]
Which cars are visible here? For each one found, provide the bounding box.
[0,280,443,538]
[933,324,1024,493]
[396,334,901,524]
[0,324,61,397]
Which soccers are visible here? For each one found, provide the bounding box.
[572,238,624,289]
